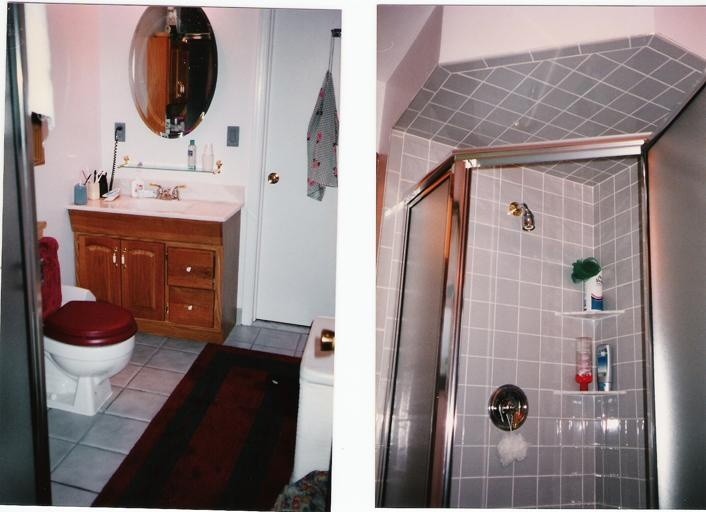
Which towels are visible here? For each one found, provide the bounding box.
[36,237,62,320]
[306,71,339,200]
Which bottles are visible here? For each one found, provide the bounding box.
[202,143,212,171]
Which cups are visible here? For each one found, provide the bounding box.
[74,185,88,205]
[87,182,101,199]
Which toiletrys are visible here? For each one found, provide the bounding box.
[187,139,197,171]
[201,141,213,172]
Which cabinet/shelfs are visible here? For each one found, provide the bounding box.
[166,212,241,345]
[146,36,191,138]
[554,309,626,397]
[68,210,166,336]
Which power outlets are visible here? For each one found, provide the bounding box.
[114,122,125,142]
[227,126,239,147]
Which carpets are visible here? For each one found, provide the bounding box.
[89,343,302,511]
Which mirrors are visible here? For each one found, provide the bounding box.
[129,6,218,140]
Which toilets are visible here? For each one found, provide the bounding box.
[35,221,137,417]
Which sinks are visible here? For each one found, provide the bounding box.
[119,198,184,212]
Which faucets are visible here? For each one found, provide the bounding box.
[161,187,170,199]
